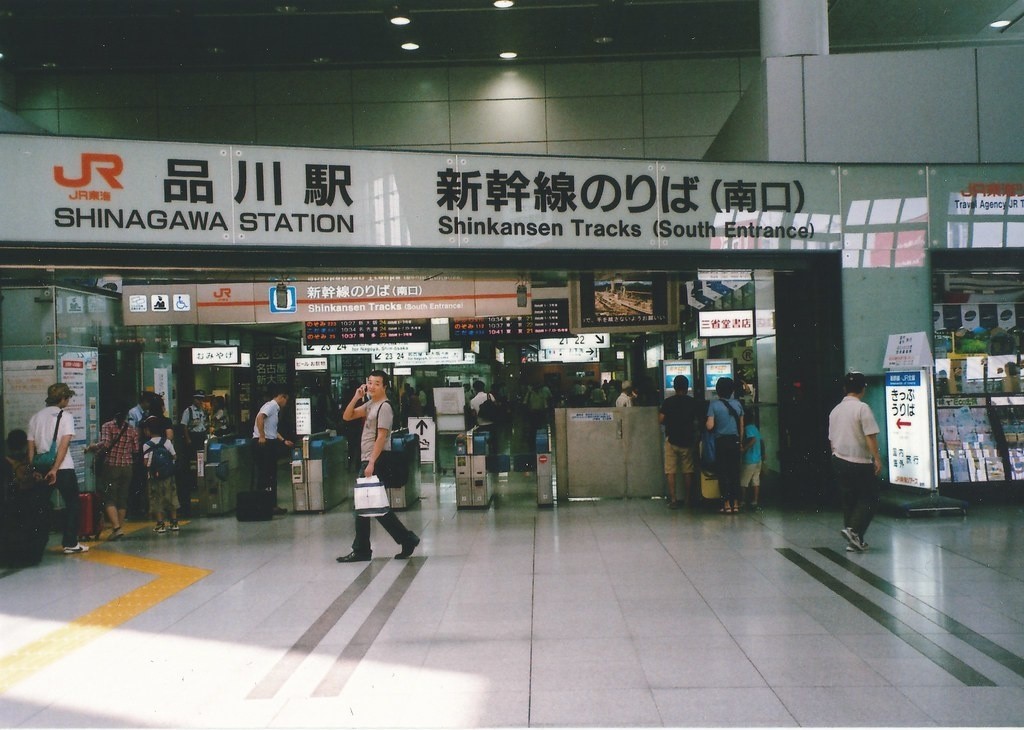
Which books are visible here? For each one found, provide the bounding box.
[937,405,1024,482]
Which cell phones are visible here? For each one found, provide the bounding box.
[364,386,367,392]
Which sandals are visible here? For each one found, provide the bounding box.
[720,507,732,513]
[734,506,740,512]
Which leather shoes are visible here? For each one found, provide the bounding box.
[395,531,420,559]
[337,550,372,562]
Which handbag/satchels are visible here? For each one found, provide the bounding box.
[478,393,497,420]
[716,434,740,453]
[96,445,108,464]
[354,475,390,517]
[380,451,409,488]
[33,452,56,477]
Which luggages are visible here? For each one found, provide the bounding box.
[237,448,274,521]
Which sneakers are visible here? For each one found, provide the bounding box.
[153,521,166,532]
[108,528,123,540]
[63,541,89,554]
[169,519,179,530]
[846,541,868,550]
[841,527,864,551]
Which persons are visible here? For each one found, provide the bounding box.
[84,401,140,541]
[740,410,761,508]
[250,391,294,515]
[704,377,745,513]
[26,383,90,554]
[209,396,226,436]
[829,372,882,554]
[658,375,702,511]
[124,390,174,442]
[400,379,436,425]
[180,389,209,450]
[460,381,474,404]
[337,370,421,564]
[469,381,497,455]
[615,380,634,407]
[143,422,180,532]
[3,429,42,520]
[491,379,614,438]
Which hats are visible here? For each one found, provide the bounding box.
[45,383,73,402]
[193,389,205,399]
[140,391,155,399]
[845,373,867,387]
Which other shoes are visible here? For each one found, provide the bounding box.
[667,502,679,508]
[273,507,287,515]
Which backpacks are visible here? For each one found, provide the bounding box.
[145,437,172,479]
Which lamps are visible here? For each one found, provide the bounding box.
[400,28,420,51]
[498,40,519,59]
[389,1,411,25]
[591,13,615,44]
